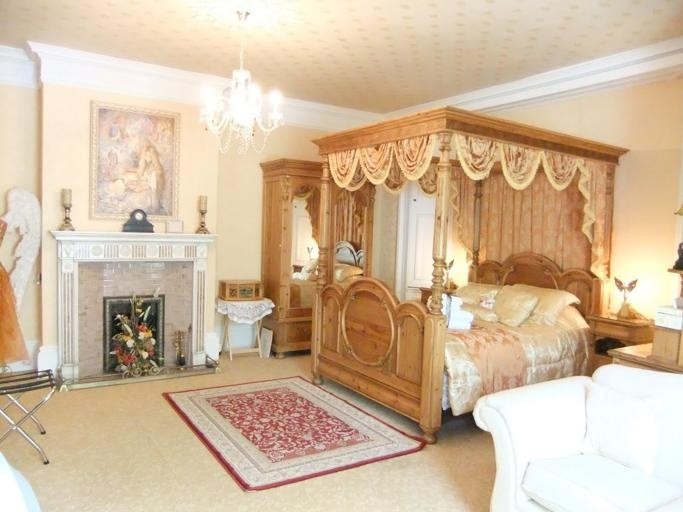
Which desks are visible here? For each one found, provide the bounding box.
[607,342,683,374]
[215,297,275,360]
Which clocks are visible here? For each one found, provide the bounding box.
[122,209,153,232]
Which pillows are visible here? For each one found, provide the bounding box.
[455,281,581,327]
[334,262,363,282]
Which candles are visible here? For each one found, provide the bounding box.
[199,196,207,210]
[63,189,72,204]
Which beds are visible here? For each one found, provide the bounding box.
[290,241,365,308]
[310,105,630,445]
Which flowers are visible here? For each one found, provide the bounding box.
[109,287,160,377]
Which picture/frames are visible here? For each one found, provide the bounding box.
[89,100,182,224]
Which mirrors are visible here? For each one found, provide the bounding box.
[290,185,366,308]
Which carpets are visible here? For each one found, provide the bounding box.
[162,376,428,494]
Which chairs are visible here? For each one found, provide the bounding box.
[472,364,683,512]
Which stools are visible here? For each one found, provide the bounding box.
[0,369,56,464]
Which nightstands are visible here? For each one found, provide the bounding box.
[419,287,452,306]
[585,313,655,377]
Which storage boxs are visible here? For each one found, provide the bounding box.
[218,279,264,300]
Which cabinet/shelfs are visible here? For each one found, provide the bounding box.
[260,158,376,359]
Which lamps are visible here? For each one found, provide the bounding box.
[199,9,285,156]
[673,203,683,270]
